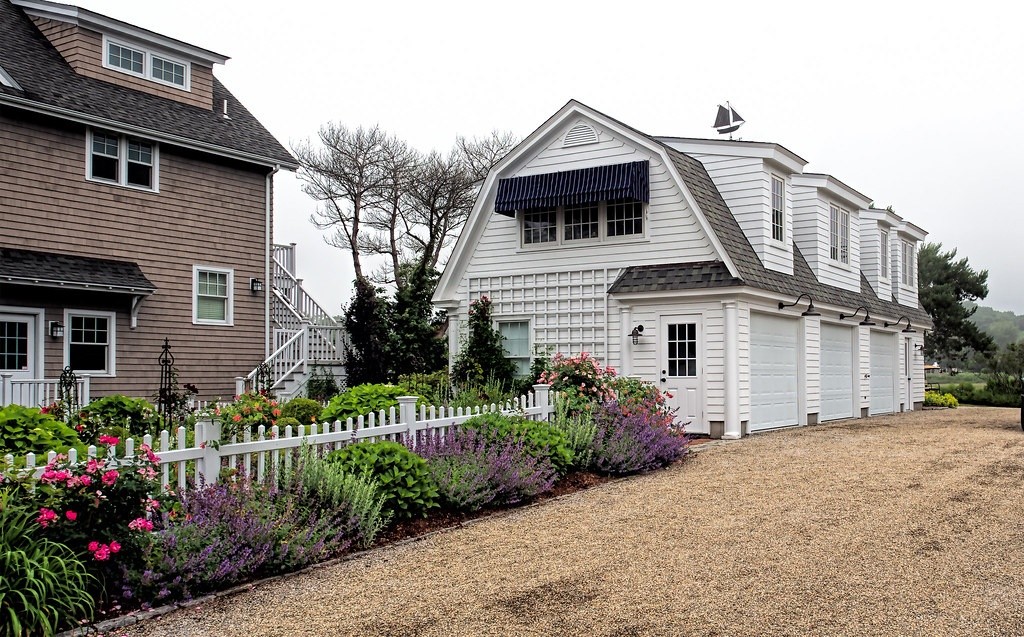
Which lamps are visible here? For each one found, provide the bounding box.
[779,294,821,316]
[840,306,876,325]
[250,278,262,291]
[49,321,66,336]
[885,315,917,332]
[915,344,927,350]
[628,325,644,345]
